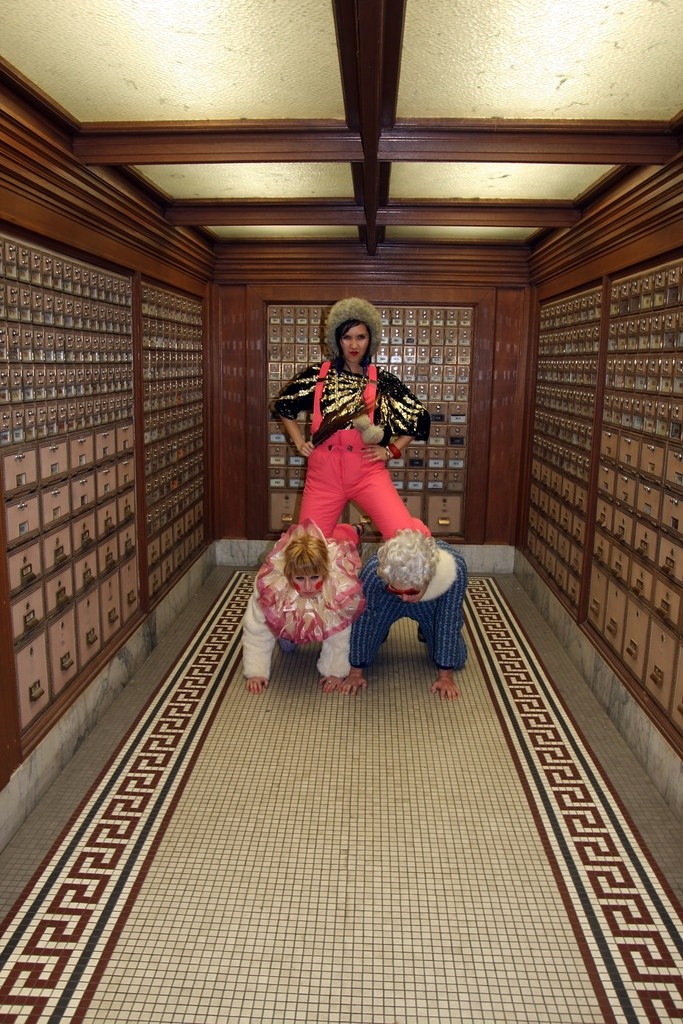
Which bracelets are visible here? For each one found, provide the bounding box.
[389,444,401,459]
[385,446,390,461]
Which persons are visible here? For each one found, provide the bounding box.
[339,528,467,701]
[274,297,430,538]
[242,519,366,694]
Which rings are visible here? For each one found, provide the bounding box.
[329,681,332,683]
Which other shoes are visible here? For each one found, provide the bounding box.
[352,523,366,558]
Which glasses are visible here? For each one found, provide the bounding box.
[385,583,420,595]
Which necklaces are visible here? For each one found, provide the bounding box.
[335,373,365,416]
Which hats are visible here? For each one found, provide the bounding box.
[326,298,381,358]
